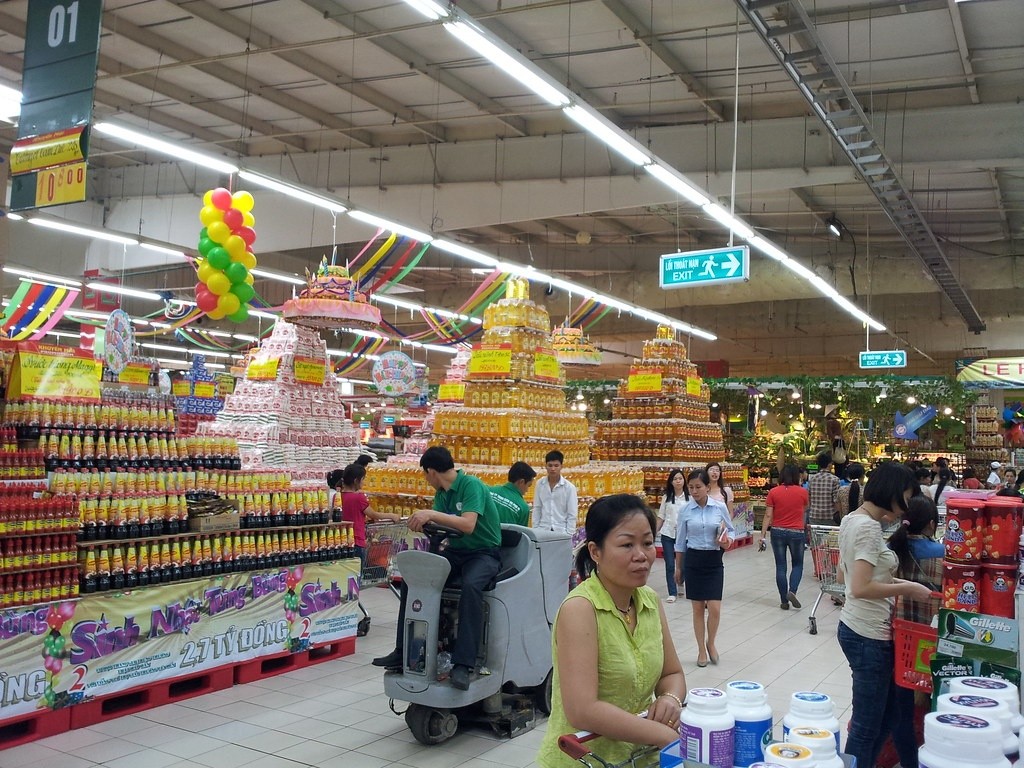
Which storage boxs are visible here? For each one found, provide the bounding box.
[660,737,857,768]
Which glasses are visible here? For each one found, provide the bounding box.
[710,468,721,473]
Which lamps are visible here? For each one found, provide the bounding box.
[93,0,887,358]
[825,219,843,237]
[575,132,592,247]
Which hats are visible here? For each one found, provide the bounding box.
[991,462,1001,469]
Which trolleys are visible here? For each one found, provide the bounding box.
[807,514,947,635]
[558,700,686,768]
[358,517,409,638]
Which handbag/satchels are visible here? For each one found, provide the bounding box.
[833,439,846,464]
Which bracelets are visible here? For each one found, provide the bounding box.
[657,693,682,710]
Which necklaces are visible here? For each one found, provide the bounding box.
[615,597,632,623]
[861,506,876,520]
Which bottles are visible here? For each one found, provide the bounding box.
[783,692,840,758]
[725,680,773,768]
[680,688,735,768]
[917,676,1024,768]
[788,726,844,768]
[748,743,818,768]
[360,273,751,529]
[0,366,355,608]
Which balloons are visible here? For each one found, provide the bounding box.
[196,187,255,323]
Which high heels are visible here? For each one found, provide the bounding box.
[706,642,719,664]
[697,649,707,666]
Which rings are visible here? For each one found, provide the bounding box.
[669,720,674,726]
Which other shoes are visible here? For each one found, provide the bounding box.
[678,585,684,597]
[372,647,403,666]
[788,591,801,608]
[781,603,789,610]
[449,664,470,690]
[667,596,677,603]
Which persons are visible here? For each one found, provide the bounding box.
[531,450,578,535]
[757,464,809,610]
[371,446,504,691]
[802,452,1024,703]
[484,460,537,547]
[836,463,933,768]
[674,470,736,667]
[656,469,691,603]
[536,493,688,768]
[704,462,734,609]
[326,455,401,570]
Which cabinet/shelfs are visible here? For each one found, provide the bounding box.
[0,420,362,721]
[198,326,362,488]
[589,340,755,539]
[361,348,646,544]
